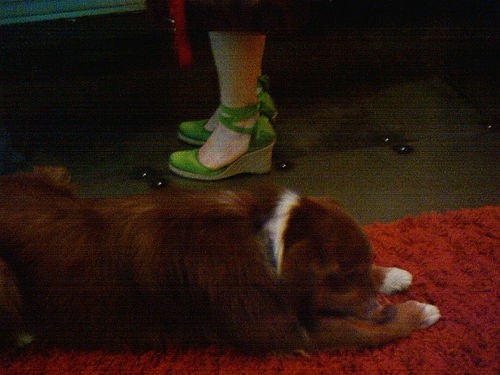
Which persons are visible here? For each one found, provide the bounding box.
[167,0,277,182]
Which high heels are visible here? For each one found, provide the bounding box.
[177,75,279,145]
[169,102,277,181]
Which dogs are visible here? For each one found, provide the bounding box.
[0,167,441,358]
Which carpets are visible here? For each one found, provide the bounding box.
[0,204,499,371]
